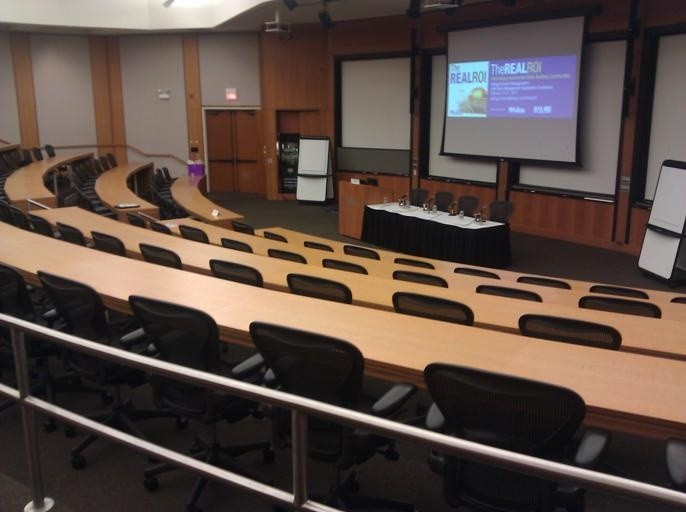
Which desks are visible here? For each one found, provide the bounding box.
[360,202,509,267]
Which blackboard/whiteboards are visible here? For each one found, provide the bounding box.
[297,136,330,177]
[296,175,328,203]
[637,227,681,282]
[646,160,686,238]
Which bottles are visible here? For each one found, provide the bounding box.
[459,210,465,222]
[431,205,437,215]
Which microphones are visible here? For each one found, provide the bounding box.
[450,201,457,208]
[402,194,408,199]
[427,195,435,203]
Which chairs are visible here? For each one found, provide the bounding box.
[458,195,480,217]
[0,229,685,511]
[409,187,427,206]
[0,141,257,257]
[435,191,453,211]
[490,201,514,223]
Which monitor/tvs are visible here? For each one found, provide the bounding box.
[367,178,377,186]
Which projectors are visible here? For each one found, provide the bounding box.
[420,0,459,11]
[264,21,291,33]
